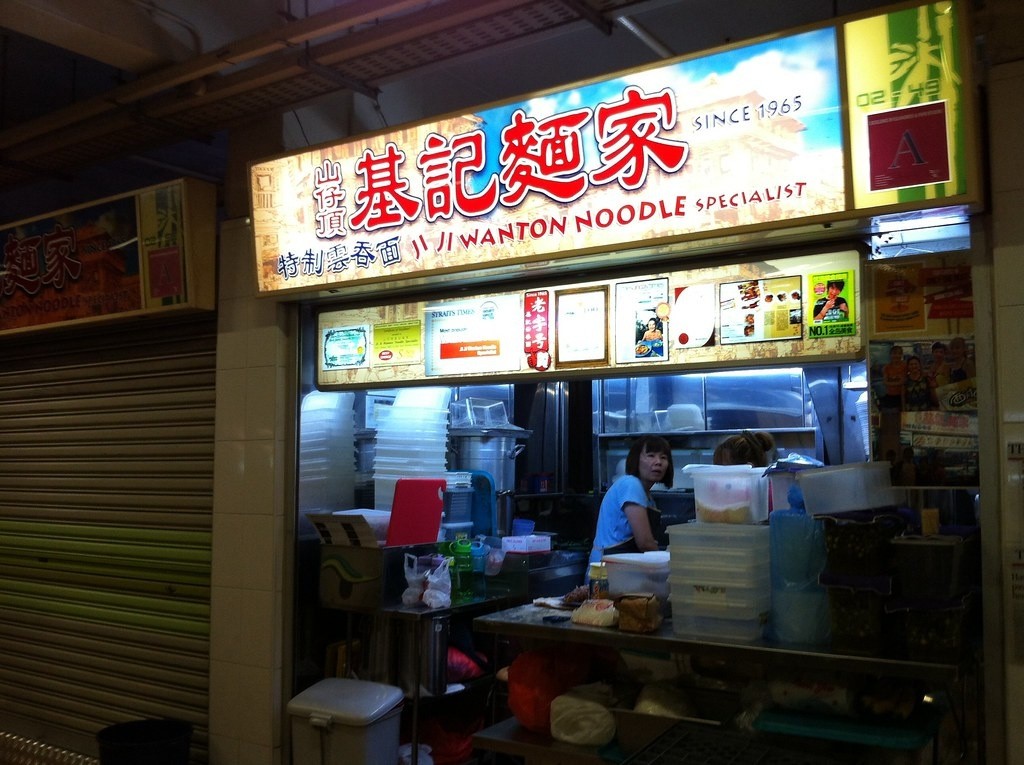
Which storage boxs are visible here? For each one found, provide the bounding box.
[307,513,386,607]
[602,460,896,643]
[299,388,475,544]
[502,535,551,553]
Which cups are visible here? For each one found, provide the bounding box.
[512,519,535,536]
[453,538,473,602]
[471,542,486,602]
[496,491,513,538]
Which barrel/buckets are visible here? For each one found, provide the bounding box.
[97,718,193,765]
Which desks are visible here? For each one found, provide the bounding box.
[473,603,979,765]
[332,594,534,765]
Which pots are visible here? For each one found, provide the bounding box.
[447,436,525,490]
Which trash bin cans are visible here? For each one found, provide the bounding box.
[95,718,192,765]
[286,676,405,765]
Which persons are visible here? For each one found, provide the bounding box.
[586,433,675,596]
[881,337,978,487]
[636,318,664,343]
[813,280,849,323]
[713,430,774,515]
